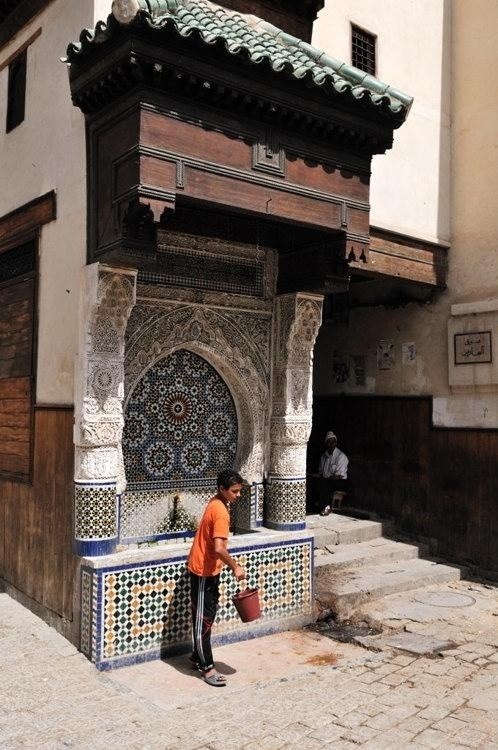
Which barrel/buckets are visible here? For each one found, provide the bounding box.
[230,578,261,622]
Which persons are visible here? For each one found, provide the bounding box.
[187,468,246,687]
[312,430,349,516]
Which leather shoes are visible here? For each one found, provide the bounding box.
[320,509,331,516]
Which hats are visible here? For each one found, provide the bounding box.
[325,431,338,442]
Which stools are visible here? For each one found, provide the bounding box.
[331,490,347,510]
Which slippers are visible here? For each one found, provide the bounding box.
[186,657,201,668]
[200,672,227,686]
